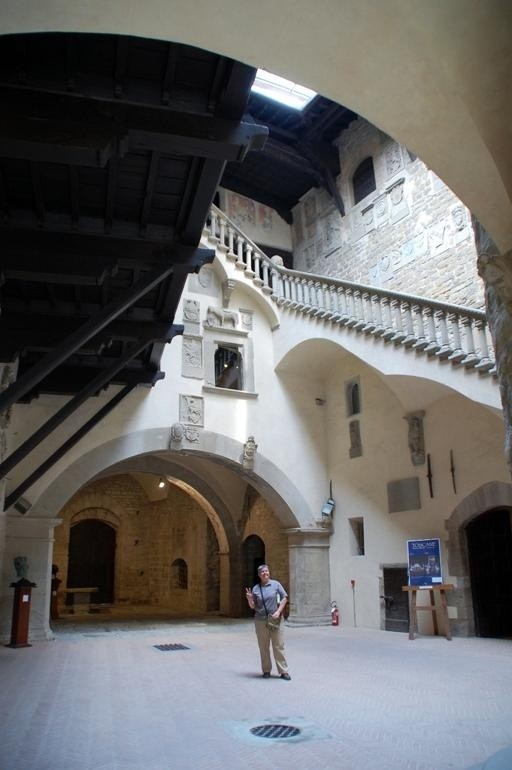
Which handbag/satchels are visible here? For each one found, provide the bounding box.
[266,613,282,631]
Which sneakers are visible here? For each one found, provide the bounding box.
[262,672,291,681]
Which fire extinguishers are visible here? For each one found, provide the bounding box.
[331,600,339,626]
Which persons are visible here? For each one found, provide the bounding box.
[243,565,292,682]
